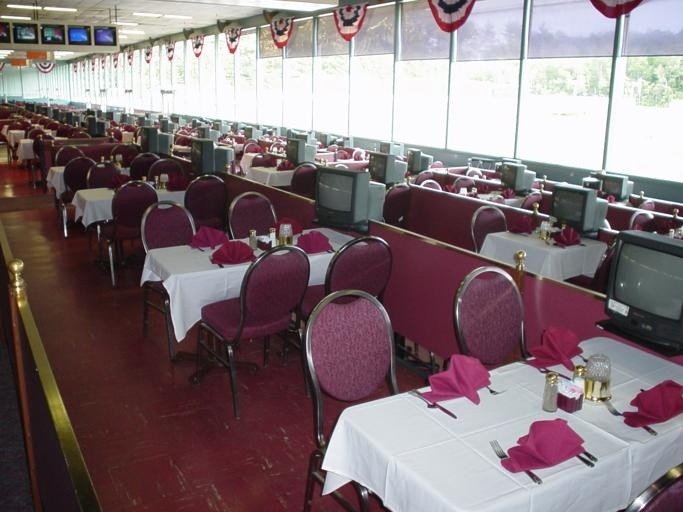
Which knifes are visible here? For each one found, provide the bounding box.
[412,389,458,420]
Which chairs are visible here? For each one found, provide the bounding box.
[302,288,403,512]
[224,121,443,239]
[442,152,683,289]
[626,464,683,512]
[192,245,311,422]
[300,237,393,324]
[451,266,530,371]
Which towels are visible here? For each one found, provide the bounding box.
[297,231,332,253]
[526,325,583,372]
[419,352,491,404]
[620,380,683,429]
[272,219,302,236]
[190,225,229,247]
[209,241,256,265]
[500,416,586,474]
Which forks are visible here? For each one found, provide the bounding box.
[607,403,657,435]
[486,386,507,395]
[488,441,542,485]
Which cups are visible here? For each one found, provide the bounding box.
[280,224,293,246]
[160,173,168,188]
[540,221,551,240]
[116,154,123,162]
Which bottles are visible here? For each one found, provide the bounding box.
[575,365,586,398]
[154,176,159,189]
[101,156,104,163]
[586,354,611,401]
[142,176,147,182]
[543,371,558,412]
[269,228,277,247]
[249,229,257,249]
[669,229,675,239]
[110,155,113,163]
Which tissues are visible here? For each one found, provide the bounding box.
[257,235,272,251]
[558,379,583,414]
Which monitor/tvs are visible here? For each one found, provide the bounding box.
[171,115,187,127]
[160,117,179,133]
[501,158,521,167]
[606,230,681,358]
[286,138,315,165]
[501,162,536,194]
[66,23,92,46]
[82,108,136,126]
[315,167,386,231]
[40,22,66,46]
[214,119,231,134]
[286,125,315,141]
[22,102,108,137]
[320,133,336,146]
[369,152,409,187]
[380,141,404,156]
[138,116,153,127]
[192,119,205,130]
[95,25,118,47]
[13,23,40,44]
[232,120,248,134]
[197,125,220,142]
[244,126,262,141]
[605,172,636,202]
[407,147,434,174]
[142,126,175,159]
[192,139,236,179]
[0,21,15,46]
[551,182,609,238]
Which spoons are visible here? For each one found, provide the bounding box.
[409,390,437,408]
